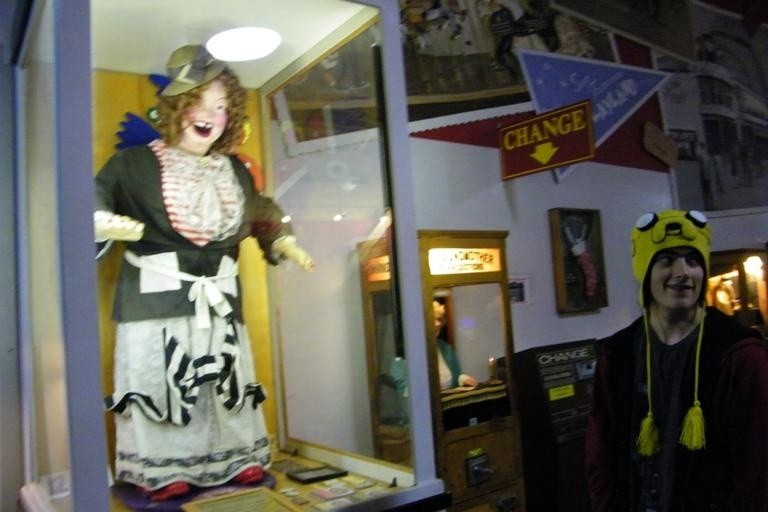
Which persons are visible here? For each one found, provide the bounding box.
[593,210,768,512]
[93,43,314,503]
[390,298,478,421]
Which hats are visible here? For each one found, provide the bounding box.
[630,209,712,307]
